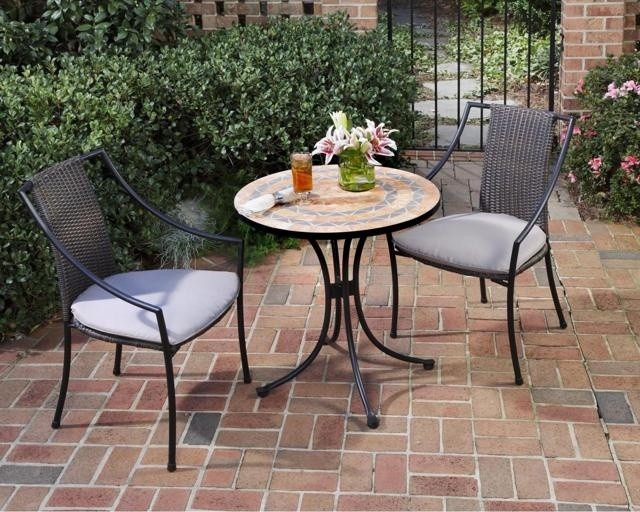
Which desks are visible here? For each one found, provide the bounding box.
[232,164,440,428]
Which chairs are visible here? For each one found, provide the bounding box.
[386,102,576,385]
[17,149,251,472]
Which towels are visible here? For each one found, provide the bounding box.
[239,186,307,216]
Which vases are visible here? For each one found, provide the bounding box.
[338,151,377,191]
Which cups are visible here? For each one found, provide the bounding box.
[290,151,313,200]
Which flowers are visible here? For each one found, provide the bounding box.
[312,109,399,166]
